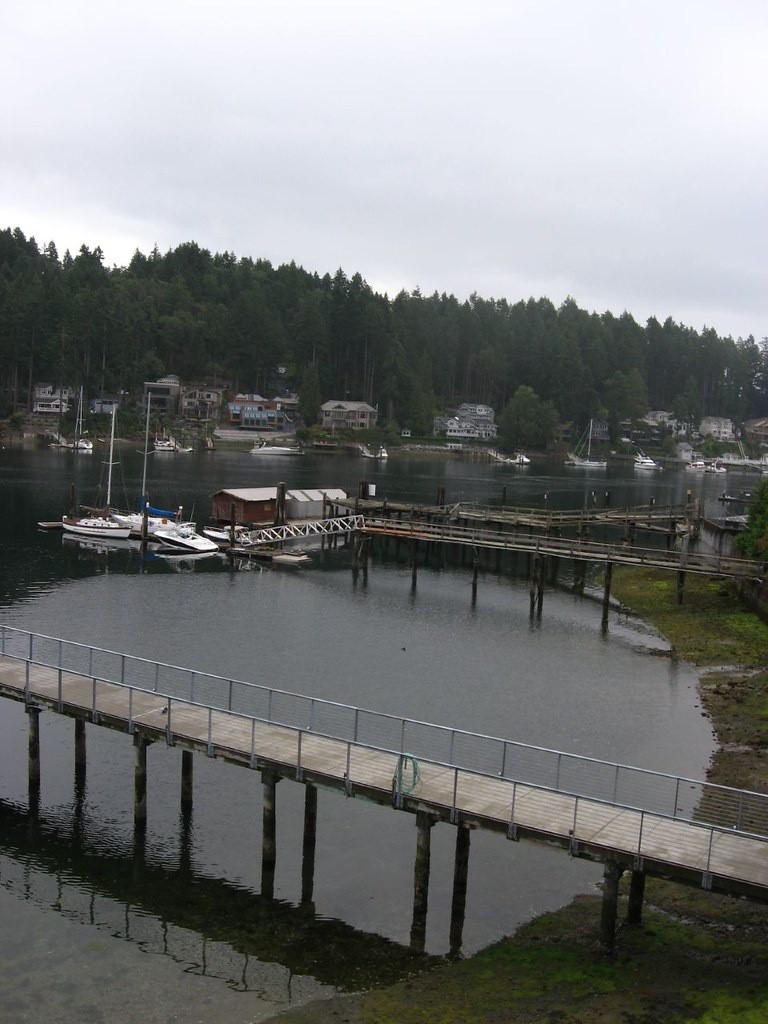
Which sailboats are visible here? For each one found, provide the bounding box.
[63,392,196,538]
[205,438,215,450]
[565,419,609,467]
[49,384,92,450]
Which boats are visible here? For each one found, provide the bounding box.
[684,460,727,473]
[249,439,305,455]
[154,436,177,450]
[633,450,661,469]
[494,454,532,465]
[154,526,218,550]
[360,445,389,459]
